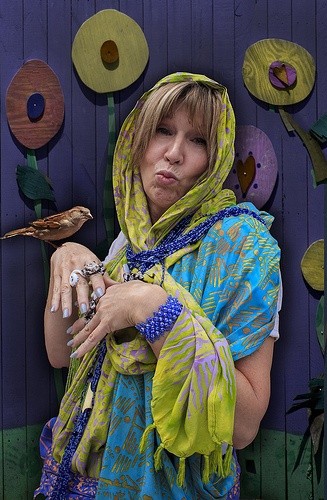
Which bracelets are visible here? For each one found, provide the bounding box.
[135,294,183,342]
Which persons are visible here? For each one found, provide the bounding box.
[35,72,283,500]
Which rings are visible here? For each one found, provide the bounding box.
[86,299,99,320]
[83,260,106,278]
[69,270,86,287]
